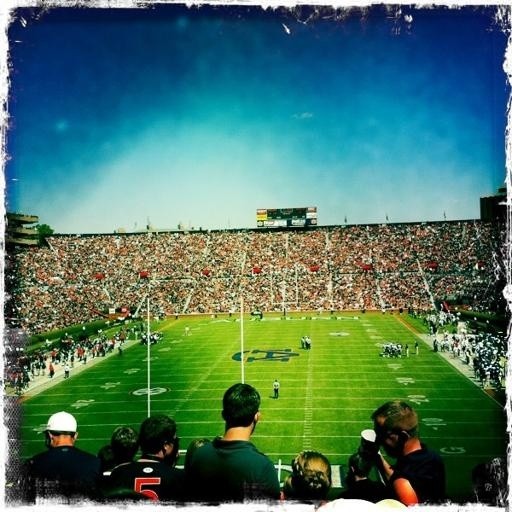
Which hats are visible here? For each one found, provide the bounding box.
[46,411,78,433]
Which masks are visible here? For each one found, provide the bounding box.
[162,438,179,461]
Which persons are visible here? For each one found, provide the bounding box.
[163,227,418,315]
[185,383,281,503]
[300,335,311,350]
[16,411,210,504]
[379,340,409,359]
[182,324,189,337]
[414,341,418,354]
[419,216,507,406]
[371,398,448,505]
[272,379,279,398]
[4,228,162,392]
[277,451,508,503]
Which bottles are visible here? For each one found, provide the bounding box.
[359,429,380,460]
